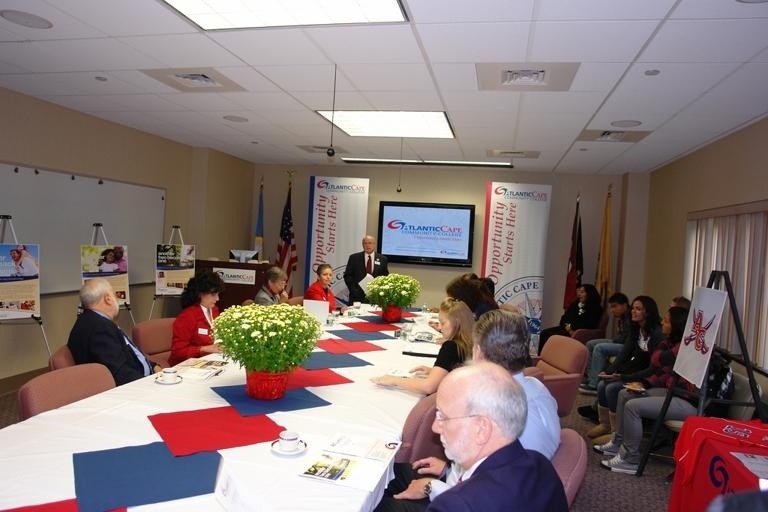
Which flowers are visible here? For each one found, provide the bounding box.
[366,272,419,309]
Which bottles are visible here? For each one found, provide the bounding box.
[327,313,333,328]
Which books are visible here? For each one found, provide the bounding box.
[170,357,228,384]
[303,431,403,498]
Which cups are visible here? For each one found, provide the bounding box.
[353,302,361,311]
[279,430,301,451]
[332,311,340,322]
[161,367,178,382]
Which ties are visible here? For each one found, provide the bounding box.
[207,308,213,324]
[366,255,372,274]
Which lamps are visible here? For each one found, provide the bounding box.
[326,62,340,155]
[396,136,403,194]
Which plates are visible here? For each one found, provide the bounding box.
[598,374,613,378]
[155,374,182,385]
[271,440,307,456]
[624,384,646,391]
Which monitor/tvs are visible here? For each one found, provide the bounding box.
[377,201,476,268]
[229,250,258,264]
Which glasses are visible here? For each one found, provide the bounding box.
[435,411,482,424]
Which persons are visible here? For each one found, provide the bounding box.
[96,246,127,272]
[369,296,477,397]
[537,282,734,474]
[255,266,288,306]
[14,244,33,269]
[10,249,37,276]
[165,271,225,366]
[373,308,560,511]
[343,234,388,304]
[98,248,119,272]
[67,277,161,388]
[424,360,567,511]
[443,270,499,316]
[302,263,336,314]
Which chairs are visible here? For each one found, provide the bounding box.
[562,301,767,484]
[1,300,591,512]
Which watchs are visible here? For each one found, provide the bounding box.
[422,482,433,496]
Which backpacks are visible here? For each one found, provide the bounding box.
[705,356,735,408]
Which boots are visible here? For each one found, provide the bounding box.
[587,403,613,439]
[592,410,623,445]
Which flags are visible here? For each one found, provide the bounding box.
[253,183,263,262]
[562,200,583,313]
[274,180,297,300]
[593,191,614,296]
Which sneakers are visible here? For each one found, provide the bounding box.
[593,431,628,458]
[577,405,600,423]
[600,444,641,475]
[578,383,599,395]
